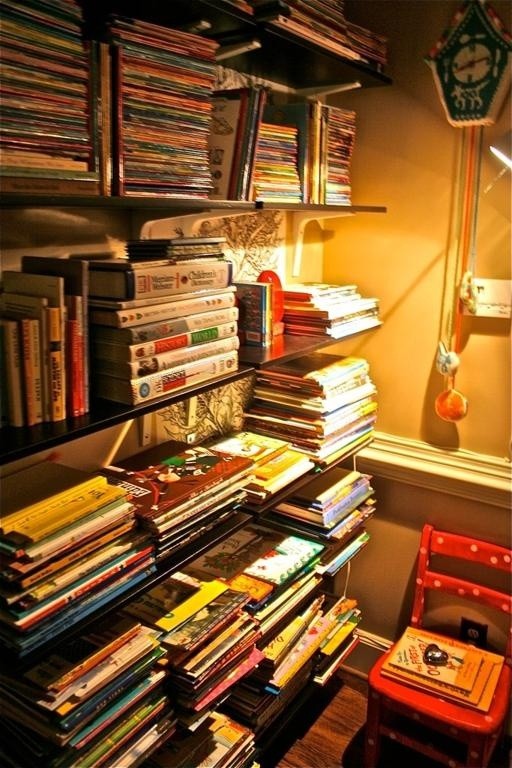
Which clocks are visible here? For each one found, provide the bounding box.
[422,0,512,422]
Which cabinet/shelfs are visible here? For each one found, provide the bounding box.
[1,0,393,768]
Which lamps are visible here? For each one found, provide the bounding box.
[489,129,512,171]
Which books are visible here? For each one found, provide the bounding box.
[380,625,506,714]
[388,632,483,693]
[380,639,495,706]
[1,2,394,767]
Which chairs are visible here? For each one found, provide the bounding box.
[366,521,512,768]
